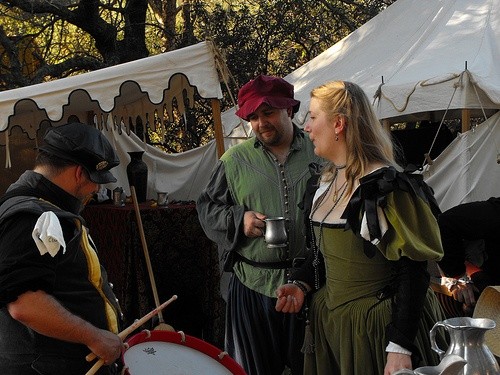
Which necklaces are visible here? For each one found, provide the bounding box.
[333,177,347,201]
[310,168,348,289]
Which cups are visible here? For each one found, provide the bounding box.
[157,191,168,206]
[259,217,287,248]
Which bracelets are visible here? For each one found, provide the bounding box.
[294,280,315,353]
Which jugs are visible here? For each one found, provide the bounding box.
[429,317,500,375]
[391,354,467,375]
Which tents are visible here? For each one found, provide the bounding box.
[0,38,225,323]
[223,0,500,219]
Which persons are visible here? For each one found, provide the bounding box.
[0,123,126,375]
[196,75,325,375]
[276,80,462,375]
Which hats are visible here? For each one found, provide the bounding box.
[235,73,300,121]
[32,121,121,184]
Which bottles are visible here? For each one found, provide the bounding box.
[127,151,148,204]
[113,187,125,207]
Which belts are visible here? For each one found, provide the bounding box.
[235,251,306,269]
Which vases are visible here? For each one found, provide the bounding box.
[127,151,148,202]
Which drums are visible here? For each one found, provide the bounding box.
[110,330,247,375]
